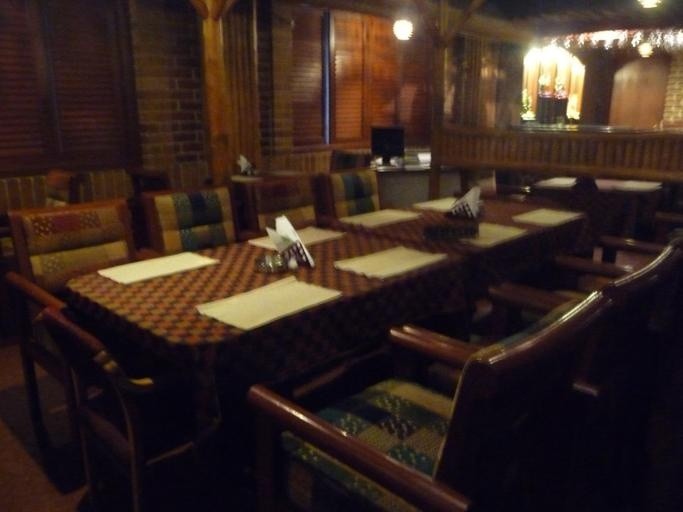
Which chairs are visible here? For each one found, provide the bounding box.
[0,168,682,511]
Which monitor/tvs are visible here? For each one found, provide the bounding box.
[370,124,405,164]
